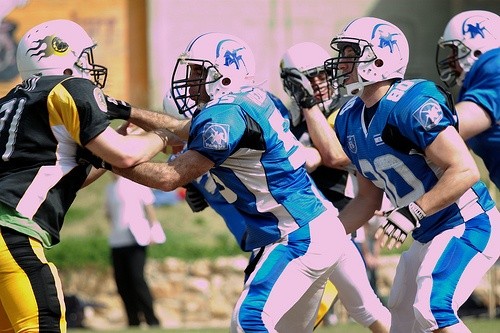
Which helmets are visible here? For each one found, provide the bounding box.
[435,9,500,89]
[16,19,108,89]
[323,17,410,103]
[280,42,339,109]
[169,32,255,120]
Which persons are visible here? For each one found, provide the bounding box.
[435,9,499,192]
[74,30,393,332]
[102,168,162,329]
[279,40,379,332]
[128,79,334,332]
[279,16,500,333]
[1,17,179,333]
[323,214,380,329]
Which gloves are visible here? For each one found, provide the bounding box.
[104,95,130,121]
[279,68,319,109]
[74,146,112,171]
